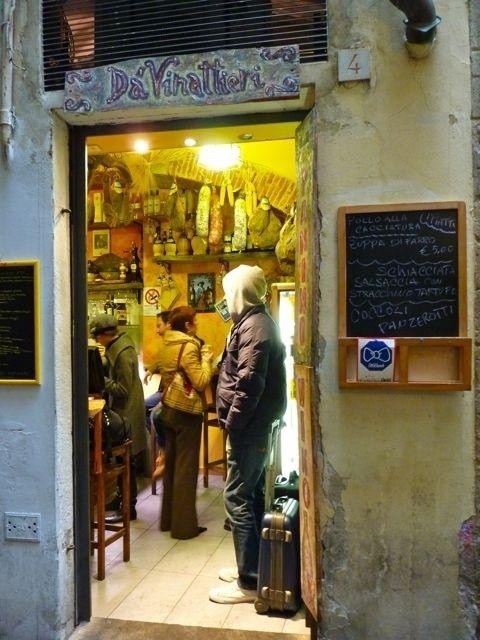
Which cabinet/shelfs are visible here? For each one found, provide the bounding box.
[86,281,143,336]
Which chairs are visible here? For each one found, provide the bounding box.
[201,378,284,487]
[89,394,134,582]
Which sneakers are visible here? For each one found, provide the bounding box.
[217,565,241,582]
[209,582,257,605]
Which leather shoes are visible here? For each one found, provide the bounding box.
[195,526,208,534]
[105,506,139,523]
[105,495,123,510]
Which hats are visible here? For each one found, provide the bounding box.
[197,281,204,285]
[90,313,117,330]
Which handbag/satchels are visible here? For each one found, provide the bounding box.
[161,341,208,414]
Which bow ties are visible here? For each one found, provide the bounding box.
[362,347,391,362]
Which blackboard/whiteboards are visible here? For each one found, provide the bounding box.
[336,200,475,337]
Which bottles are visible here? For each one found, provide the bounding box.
[103,295,112,315]
[166,229,177,256]
[161,231,168,255]
[154,189,160,215]
[153,226,163,257]
[148,190,154,214]
[110,295,117,313]
[143,193,148,215]
[129,250,140,282]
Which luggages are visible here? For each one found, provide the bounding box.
[253,420,301,615]
[273,469,299,500]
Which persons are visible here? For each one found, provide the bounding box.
[209,265,286,604]
[89,313,146,524]
[141,311,175,476]
[159,306,212,541]
[192,280,209,308]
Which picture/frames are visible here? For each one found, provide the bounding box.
[92,229,111,258]
[186,272,215,312]
